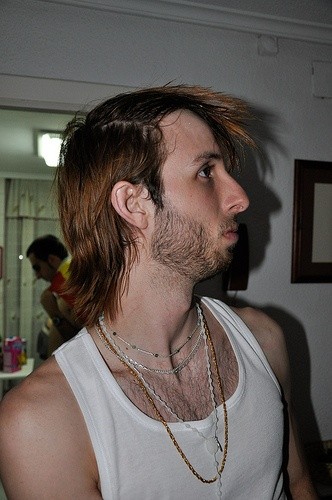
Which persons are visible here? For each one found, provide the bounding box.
[25,234,85,342]
[0,78,318,500]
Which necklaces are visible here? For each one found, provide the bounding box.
[94,300,228,500]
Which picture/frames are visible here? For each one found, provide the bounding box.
[291,159,332,284]
